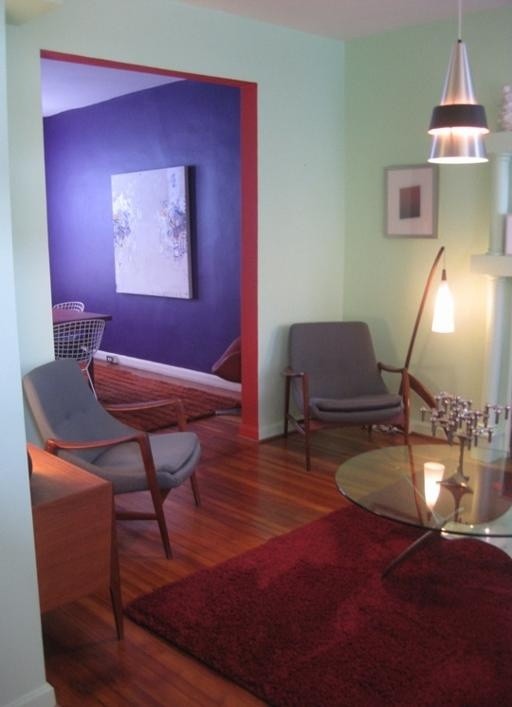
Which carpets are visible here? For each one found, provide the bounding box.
[80,362,240,432]
[124,459,512,707]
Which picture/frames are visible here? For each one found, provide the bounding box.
[384,163,439,239]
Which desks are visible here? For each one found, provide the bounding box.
[27,441,123,639]
[52,308,112,393]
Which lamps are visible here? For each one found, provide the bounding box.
[399,246,456,394]
[427,0,491,165]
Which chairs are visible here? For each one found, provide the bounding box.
[22,357,202,559]
[52,301,105,401]
[280,322,411,471]
